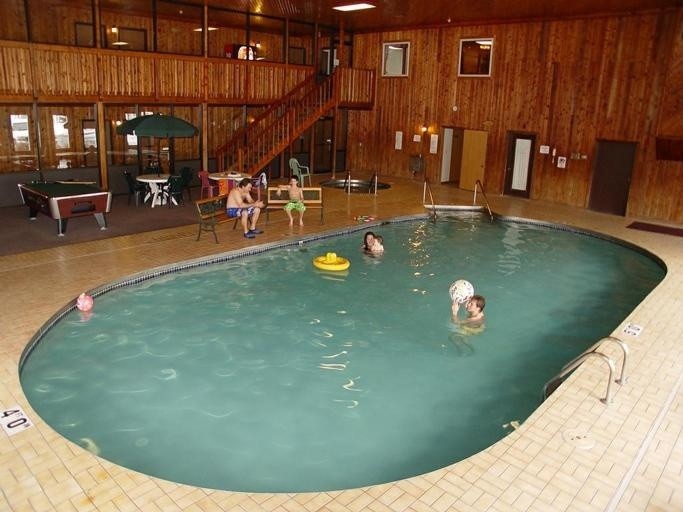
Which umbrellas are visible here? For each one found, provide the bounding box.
[115,111,200,177]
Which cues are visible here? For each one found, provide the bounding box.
[32,181,96,184]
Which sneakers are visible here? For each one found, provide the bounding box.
[244,232,255,238]
[249,228,264,234]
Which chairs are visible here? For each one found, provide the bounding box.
[122,159,268,210]
[288,157,312,187]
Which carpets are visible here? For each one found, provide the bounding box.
[0,185,310,258]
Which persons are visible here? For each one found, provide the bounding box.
[225,177,264,239]
[360,233,375,250]
[449,295,487,357]
[370,236,385,253]
[276,175,304,227]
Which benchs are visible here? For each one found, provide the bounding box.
[192,192,266,244]
[266,186,326,225]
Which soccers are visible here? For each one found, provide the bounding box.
[76,296,94,311]
[450,280,473,304]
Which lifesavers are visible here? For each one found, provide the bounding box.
[313,253,350,270]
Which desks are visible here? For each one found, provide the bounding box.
[17,179,112,238]
